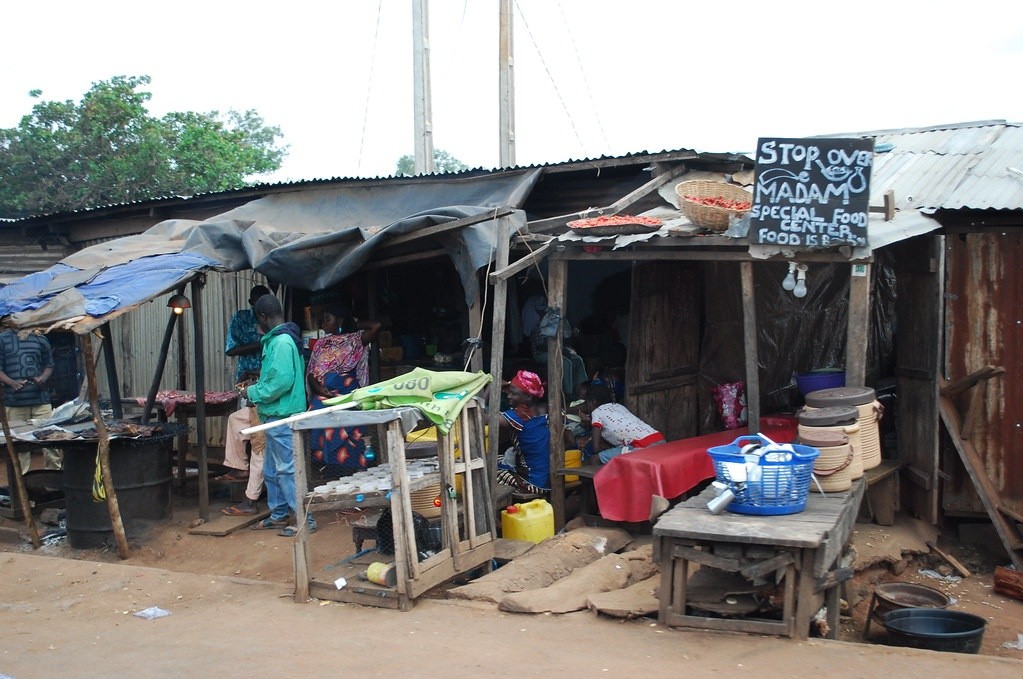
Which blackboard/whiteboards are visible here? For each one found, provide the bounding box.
[748,138,874,246]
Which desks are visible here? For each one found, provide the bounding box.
[594,411,910,637]
[103,389,239,478]
[348,483,516,553]
[0,411,158,519]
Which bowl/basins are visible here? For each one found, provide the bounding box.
[871,582,987,656]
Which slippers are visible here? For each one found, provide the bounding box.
[221,505,252,516]
[214,474,236,480]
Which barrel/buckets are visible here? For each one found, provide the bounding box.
[397,444,451,519]
[63,436,172,550]
[796,372,846,397]
[796,387,881,493]
[563,450,583,482]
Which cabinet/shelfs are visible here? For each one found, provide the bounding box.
[289,399,499,611]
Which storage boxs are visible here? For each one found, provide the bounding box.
[302,304,332,350]
[378,330,404,362]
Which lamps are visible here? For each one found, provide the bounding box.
[166,287,192,314]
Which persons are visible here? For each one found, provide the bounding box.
[590,343,627,402]
[0,325,64,475]
[216,406,266,516]
[580,384,666,466]
[225,285,270,383]
[233,295,318,537]
[522,280,588,395]
[306,303,382,471]
[484,369,575,495]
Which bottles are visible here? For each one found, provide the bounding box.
[501,497,555,549]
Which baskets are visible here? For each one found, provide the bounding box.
[707,436,820,515]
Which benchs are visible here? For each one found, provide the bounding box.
[513,465,605,515]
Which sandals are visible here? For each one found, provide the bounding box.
[279,523,316,537]
[250,519,290,530]
